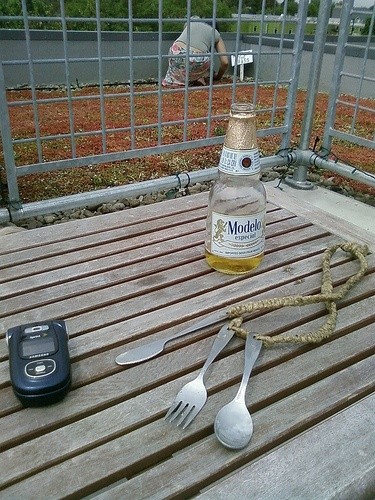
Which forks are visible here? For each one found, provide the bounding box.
[163,317,244,430]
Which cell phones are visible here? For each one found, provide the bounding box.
[6,320,71,407]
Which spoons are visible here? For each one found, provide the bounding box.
[214,331,264,449]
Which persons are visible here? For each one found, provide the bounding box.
[162,16,228,88]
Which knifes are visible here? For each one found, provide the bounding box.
[115,309,229,365]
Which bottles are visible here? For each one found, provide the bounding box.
[204,101,266,275]
